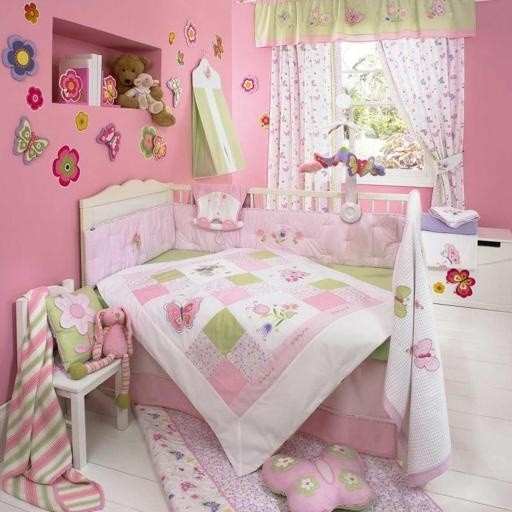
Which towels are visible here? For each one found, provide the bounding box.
[431,200,481,231]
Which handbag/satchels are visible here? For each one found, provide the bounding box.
[190,57,245,182]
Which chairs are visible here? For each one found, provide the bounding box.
[17,279,133,469]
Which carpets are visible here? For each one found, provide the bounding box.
[134,405,449,512]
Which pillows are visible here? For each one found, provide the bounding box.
[262,444,379,511]
[46,286,108,371]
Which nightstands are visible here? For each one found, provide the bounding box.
[414,223,511,314]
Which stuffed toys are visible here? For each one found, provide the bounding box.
[124,70,164,113]
[110,51,177,128]
[71,305,135,405]
[294,149,388,179]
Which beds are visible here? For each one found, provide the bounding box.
[80,179,452,489]
